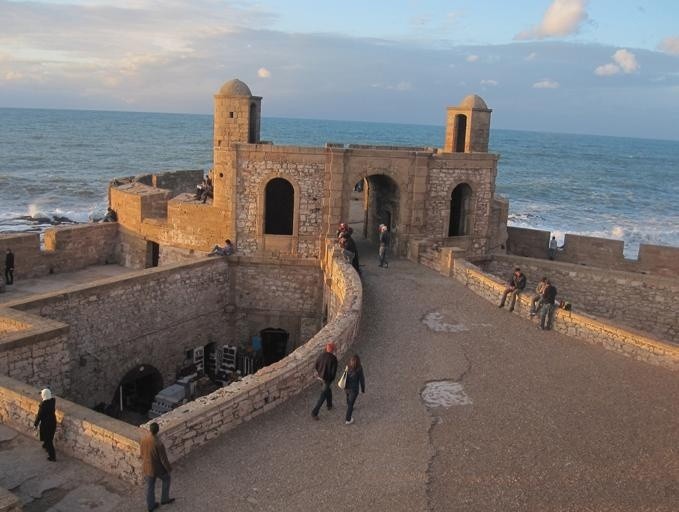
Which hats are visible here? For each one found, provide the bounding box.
[326,342,336,352]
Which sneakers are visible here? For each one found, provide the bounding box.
[47,457,56,462]
[327,407,332,410]
[498,304,552,331]
[345,417,354,424]
[310,412,319,420]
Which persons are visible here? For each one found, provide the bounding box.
[528,276,548,317]
[140,422,175,512]
[336,223,361,274]
[344,354,365,425]
[310,342,338,421]
[537,280,558,330]
[496,267,527,312]
[5,248,14,285]
[548,236,557,260]
[377,224,391,267]
[104,207,115,222]
[207,239,233,257]
[31,388,57,461]
[193,175,213,204]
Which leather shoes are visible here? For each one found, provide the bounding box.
[378,262,389,268]
[148,502,158,512]
[161,498,175,505]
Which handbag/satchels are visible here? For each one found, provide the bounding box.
[338,372,347,389]
[559,301,571,310]
